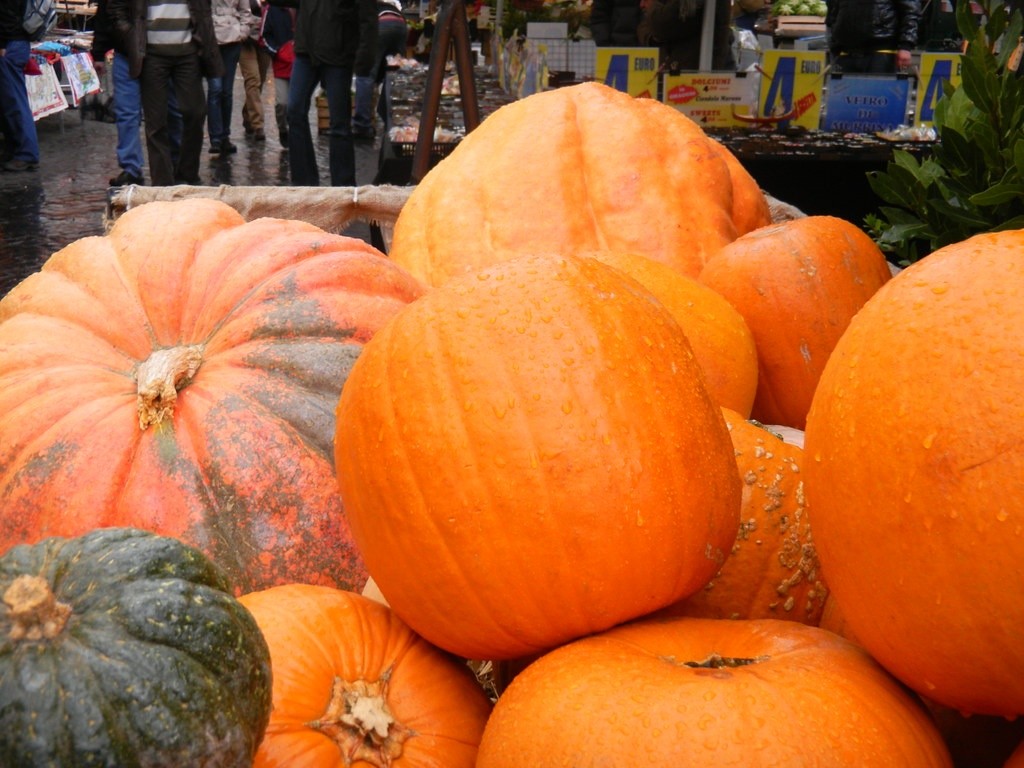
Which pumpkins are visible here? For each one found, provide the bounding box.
[0,82,1024,768]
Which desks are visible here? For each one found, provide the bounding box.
[372,66,513,186]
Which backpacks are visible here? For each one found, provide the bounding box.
[23,0,58,42]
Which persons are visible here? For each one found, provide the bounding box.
[208,0,254,153]
[107,0,225,187]
[89,0,183,187]
[590,0,764,70]
[0,0,40,171]
[825,0,922,80]
[261,0,299,149]
[239,0,271,140]
[266,0,379,186]
[351,0,407,138]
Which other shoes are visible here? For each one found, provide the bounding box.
[255,129,265,140]
[4,160,37,170]
[245,126,254,133]
[207,137,237,153]
[280,131,289,148]
[352,126,375,138]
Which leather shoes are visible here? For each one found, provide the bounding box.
[109,172,144,187]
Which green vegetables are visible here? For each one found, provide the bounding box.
[770,0,828,16]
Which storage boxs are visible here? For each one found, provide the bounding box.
[315,92,379,134]
[390,140,456,161]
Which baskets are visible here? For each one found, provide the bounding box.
[391,141,459,163]
